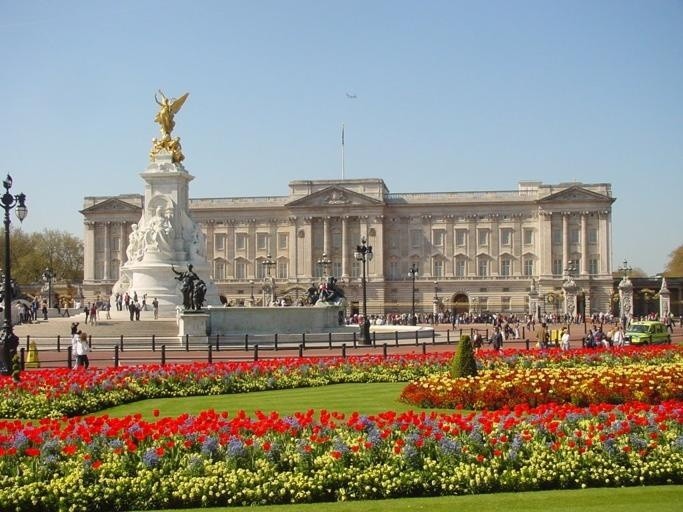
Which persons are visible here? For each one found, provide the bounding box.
[71,322,79,340]
[275,296,308,306]
[153,92,170,133]
[71,331,83,370]
[148,205,172,255]
[172,263,200,310]
[16,291,159,326]
[126,224,141,261]
[305,276,345,305]
[77,333,92,370]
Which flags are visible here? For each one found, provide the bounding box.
[341,127,345,144]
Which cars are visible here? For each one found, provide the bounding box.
[622,320,671,346]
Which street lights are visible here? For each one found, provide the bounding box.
[353,241,374,347]
[408,266,419,327]
[42,266,57,308]
[1,174,28,368]
